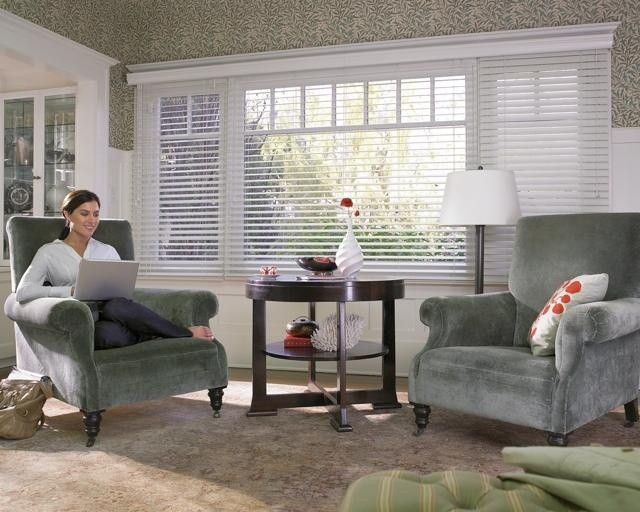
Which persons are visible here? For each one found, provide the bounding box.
[16,189,216,348]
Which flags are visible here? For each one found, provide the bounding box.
[333,218,364,278]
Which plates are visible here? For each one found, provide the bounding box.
[2,86,78,264]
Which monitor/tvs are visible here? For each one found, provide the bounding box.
[284,333,313,348]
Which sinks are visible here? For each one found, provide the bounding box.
[6,217,228,447]
[407,212,640,450]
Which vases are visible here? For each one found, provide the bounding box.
[340,197,360,219]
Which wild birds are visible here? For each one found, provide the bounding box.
[76,260,141,302]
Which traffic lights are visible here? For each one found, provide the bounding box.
[528,272,609,358]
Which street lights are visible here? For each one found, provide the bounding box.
[0,376,54,441]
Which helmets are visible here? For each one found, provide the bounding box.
[252,274,280,279]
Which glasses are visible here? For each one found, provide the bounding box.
[436,166,521,292]
